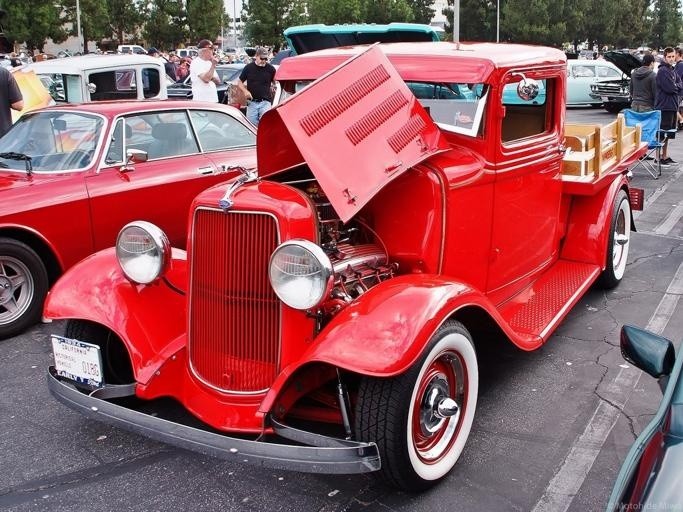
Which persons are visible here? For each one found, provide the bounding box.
[190,39,221,102]
[11,47,278,81]
[238,48,277,125]
[0,66,23,139]
[629,47,683,167]
[593,51,606,60]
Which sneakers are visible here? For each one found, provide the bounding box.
[639,154,654,161]
[654,157,678,168]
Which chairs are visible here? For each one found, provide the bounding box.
[623,109,663,179]
[106,121,188,163]
[500,111,542,143]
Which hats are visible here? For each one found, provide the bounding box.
[197,40,213,49]
[641,55,654,66]
[146,47,160,55]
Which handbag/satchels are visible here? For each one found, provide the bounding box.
[227,82,254,106]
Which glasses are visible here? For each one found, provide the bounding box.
[260,57,270,60]
